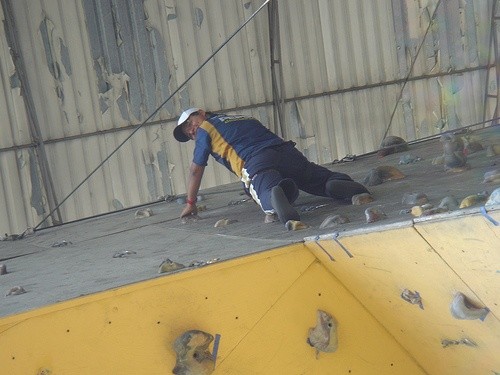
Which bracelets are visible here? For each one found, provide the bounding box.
[186,197,198,205]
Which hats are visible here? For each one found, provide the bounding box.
[172,106,203,144]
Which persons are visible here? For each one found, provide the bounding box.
[172,106,370,223]
